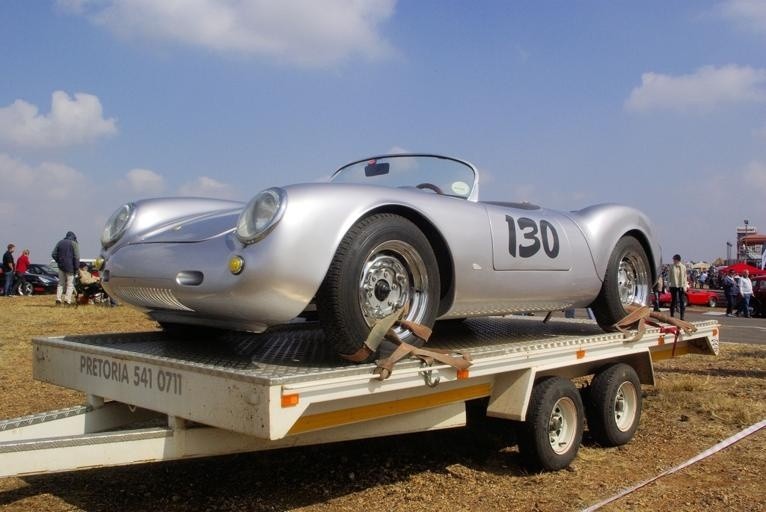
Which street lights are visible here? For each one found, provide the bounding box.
[744,219,749,263]
[727,242,733,265]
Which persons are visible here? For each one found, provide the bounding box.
[653,254,766,321]
[50,231,79,304]
[79,262,118,307]
[9,249,30,296]
[2,244,15,296]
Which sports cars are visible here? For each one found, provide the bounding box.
[94,153,664,364]
[649,284,720,308]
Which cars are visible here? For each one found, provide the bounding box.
[736,275,766,317]
[0,258,100,297]
